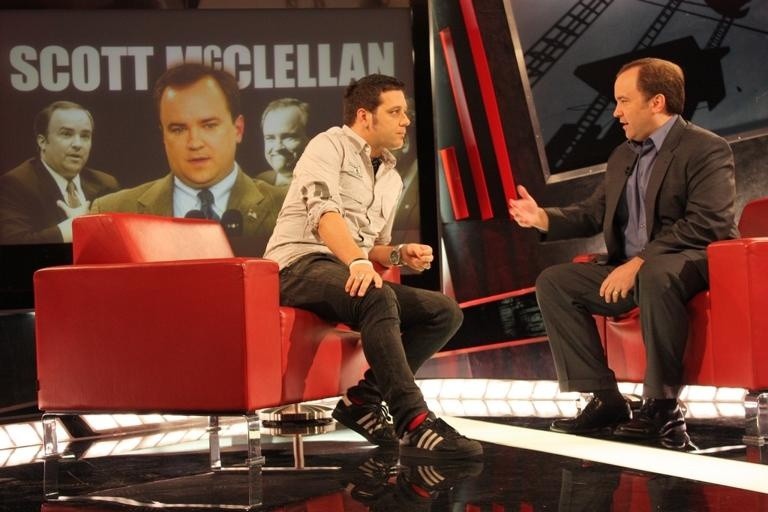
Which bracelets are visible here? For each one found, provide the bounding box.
[344,256,376,272]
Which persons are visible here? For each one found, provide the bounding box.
[338,445,484,511]
[505,57,739,453]
[88,60,286,259]
[556,460,712,511]
[251,96,310,187]
[1,99,121,245]
[385,97,421,244]
[261,76,482,456]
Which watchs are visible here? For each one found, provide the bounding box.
[389,242,409,271]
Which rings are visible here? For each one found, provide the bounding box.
[612,290,620,296]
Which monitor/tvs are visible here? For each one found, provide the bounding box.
[0,0,443,310]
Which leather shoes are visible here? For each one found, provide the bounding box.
[549,394,635,434]
[611,396,687,440]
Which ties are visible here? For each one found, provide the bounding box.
[67,181,81,209]
[197,188,215,219]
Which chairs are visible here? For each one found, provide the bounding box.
[570,195,768,446]
[33,212,403,467]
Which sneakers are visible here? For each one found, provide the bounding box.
[330,386,400,447]
[399,410,484,461]
